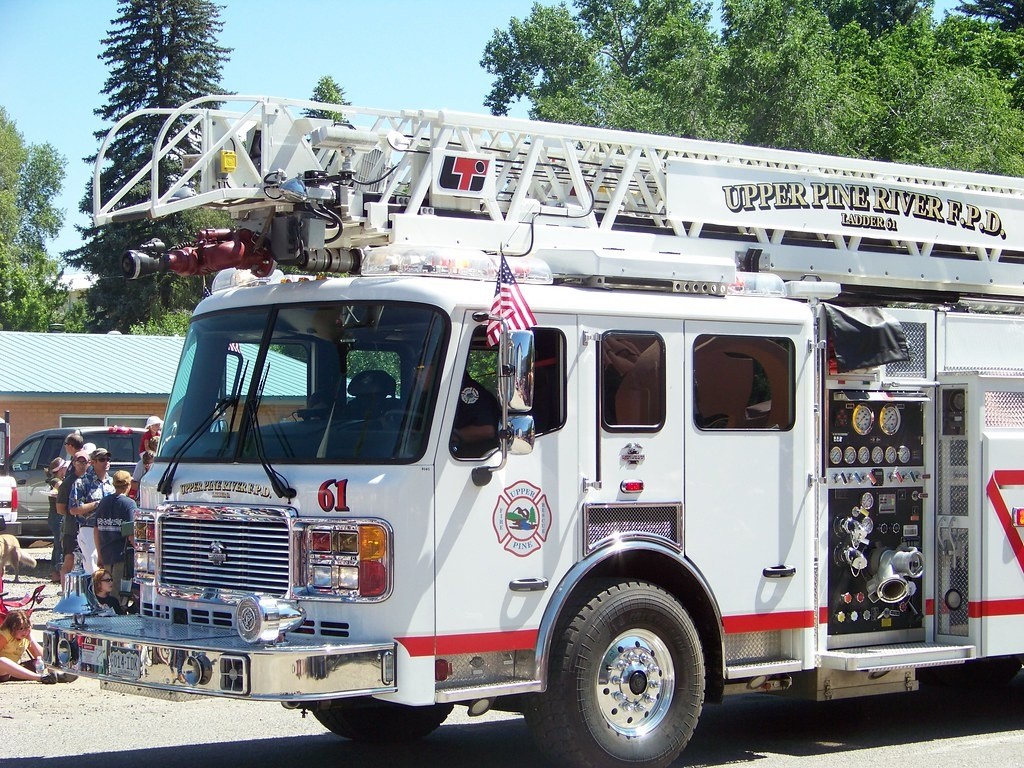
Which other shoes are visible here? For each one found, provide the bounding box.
[57,672,78,683]
[41,671,56,684]
[57,592,64,596]
[52,580,61,584]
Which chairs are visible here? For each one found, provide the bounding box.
[496,352,554,432]
[339,370,401,430]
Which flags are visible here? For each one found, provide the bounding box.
[203,284,243,354]
[486,253,536,348]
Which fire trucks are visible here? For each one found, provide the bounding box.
[37,89,1024,768]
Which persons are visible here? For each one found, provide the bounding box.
[0,409,226,686]
[409,340,502,445]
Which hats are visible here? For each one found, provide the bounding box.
[83,442,97,454]
[113,470,134,486]
[92,448,111,458]
[50,456,71,472]
[73,451,89,464]
[144,416,164,429]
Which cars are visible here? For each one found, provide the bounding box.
[0,426,153,548]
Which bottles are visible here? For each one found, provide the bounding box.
[35,657,44,674]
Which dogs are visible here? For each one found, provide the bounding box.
[0,533,37,584]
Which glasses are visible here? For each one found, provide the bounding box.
[64,442,68,445]
[412,365,426,373]
[99,578,113,582]
[97,458,110,462]
[16,626,31,631]
